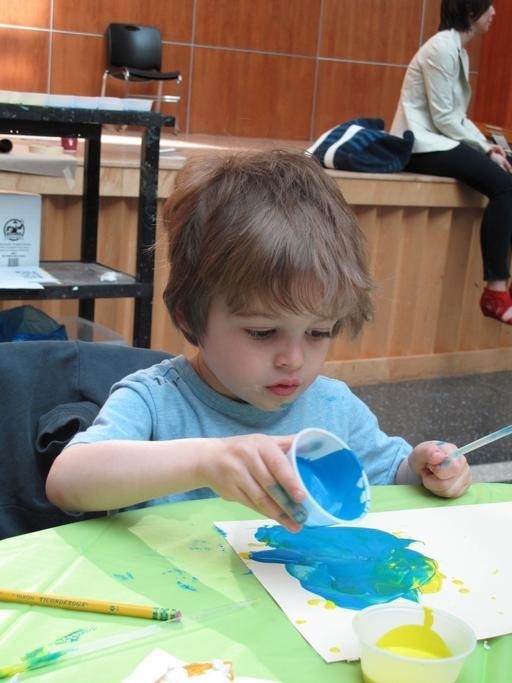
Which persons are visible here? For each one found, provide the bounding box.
[38,145,474,536]
[387,0,512,333]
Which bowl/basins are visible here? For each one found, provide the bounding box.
[351,602,476,683]
[282,427,371,529]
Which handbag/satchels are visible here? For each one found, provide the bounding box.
[301,117,414,172]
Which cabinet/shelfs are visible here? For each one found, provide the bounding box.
[0,102,176,351]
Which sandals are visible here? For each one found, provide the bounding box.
[479,288,512,326]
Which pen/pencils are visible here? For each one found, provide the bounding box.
[445,426,512,462]
[0,591,181,620]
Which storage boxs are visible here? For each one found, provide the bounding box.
[1,317,128,345]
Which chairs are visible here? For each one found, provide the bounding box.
[101,22,182,136]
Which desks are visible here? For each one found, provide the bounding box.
[0,481,512,683]
